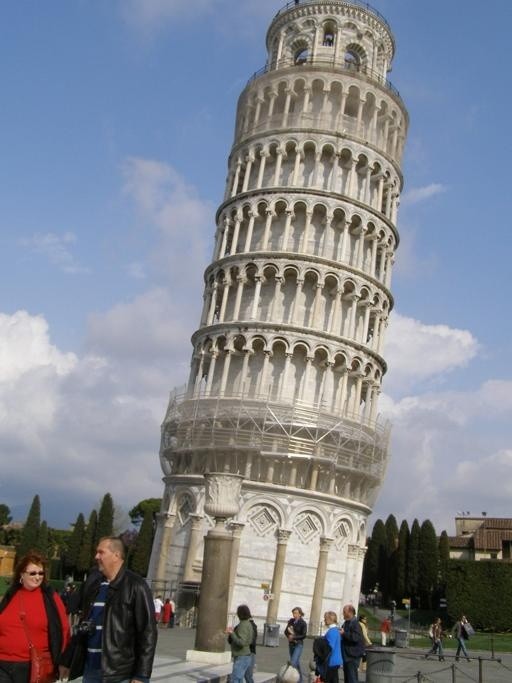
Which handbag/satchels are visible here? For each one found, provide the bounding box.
[346,641,365,658]
[30,648,57,683]
[463,623,476,636]
[60,631,79,669]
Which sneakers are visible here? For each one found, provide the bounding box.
[455,657,471,662]
[439,656,443,661]
[425,654,428,658]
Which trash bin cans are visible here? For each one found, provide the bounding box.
[395,630,407,648]
[263,623,280,647]
[365,648,397,683]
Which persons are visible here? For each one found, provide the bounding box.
[358,614,372,672]
[223,604,254,682]
[312,610,342,682]
[153,595,176,628]
[380,615,392,646]
[339,604,367,682]
[58,535,158,683]
[1,551,71,683]
[424,616,449,661]
[227,614,257,682]
[449,615,473,661]
[284,606,307,682]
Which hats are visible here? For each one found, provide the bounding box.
[238,607,251,618]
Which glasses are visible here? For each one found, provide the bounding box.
[30,571,44,575]
